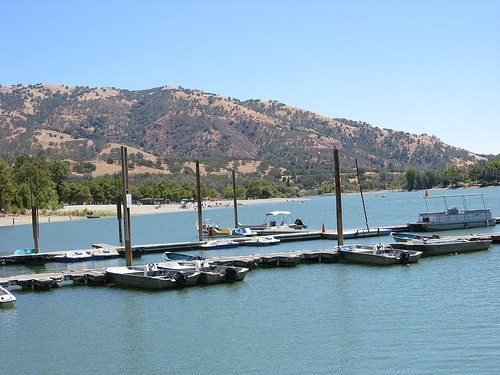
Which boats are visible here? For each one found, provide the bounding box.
[0,285,17,305]
[55,245,121,263]
[406,193,496,231]
[389,231,442,243]
[150,262,250,285]
[389,236,495,258]
[162,251,204,262]
[334,244,425,266]
[196,208,310,249]
[13,249,36,255]
[103,263,202,290]
